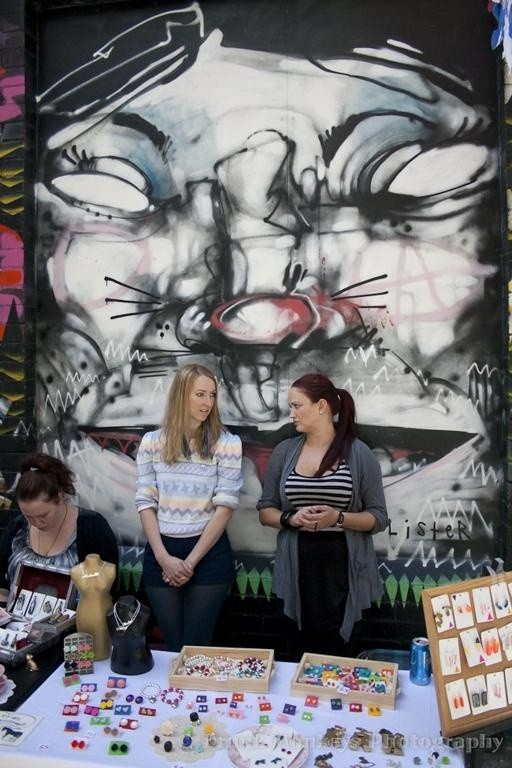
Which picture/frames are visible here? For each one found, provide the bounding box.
[423,569,512,737]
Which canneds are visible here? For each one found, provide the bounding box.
[409,636,431,686]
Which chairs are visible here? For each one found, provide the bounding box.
[357,649,410,670]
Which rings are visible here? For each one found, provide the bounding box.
[312,520,318,532]
[180,575,189,582]
[166,578,171,582]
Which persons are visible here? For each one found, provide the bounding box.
[135,364,244,652]
[0,454,120,637]
[255,373,390,664]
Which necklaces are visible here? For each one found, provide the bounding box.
[79,560,107,579]
[112,604,140,631]
[180,435,196,460]
[115,599,141,626]
[38,501,68,557]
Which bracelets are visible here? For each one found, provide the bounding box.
[160,687,185,709]
[330,511,345,529]
[179,654,266,681]
[140,682,163,704]
[279,508,303,531]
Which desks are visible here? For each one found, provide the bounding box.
[0,646,466,768]
